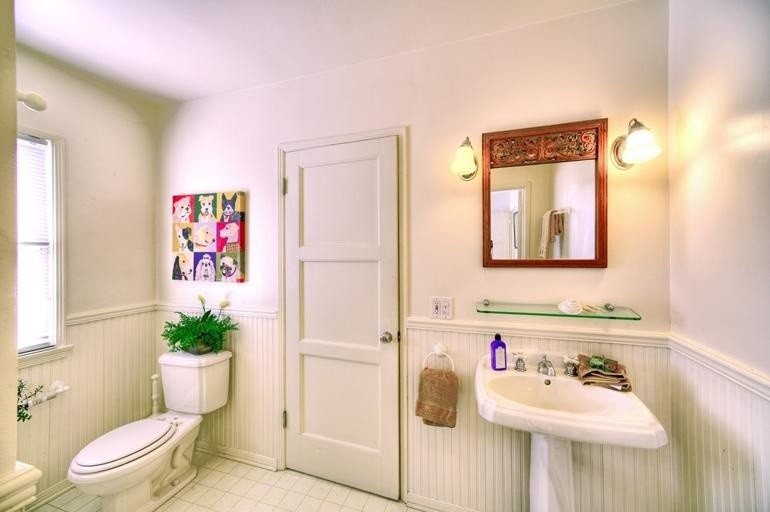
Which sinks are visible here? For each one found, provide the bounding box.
[472,363,670,451]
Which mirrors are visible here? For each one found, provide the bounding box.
[481,117,607,268]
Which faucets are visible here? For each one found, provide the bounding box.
[536,359,557,377]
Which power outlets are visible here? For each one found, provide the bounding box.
[428,297,453,321]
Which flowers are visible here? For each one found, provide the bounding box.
[161,293,240,354]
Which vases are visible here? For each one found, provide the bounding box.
[188,343,213,355]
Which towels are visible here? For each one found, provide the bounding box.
[415,368,459,429]
[578,353,632,393]
[538,209,563,258]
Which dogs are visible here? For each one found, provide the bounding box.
[173,193,244,283]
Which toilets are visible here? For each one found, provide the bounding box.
[64,343,233,512]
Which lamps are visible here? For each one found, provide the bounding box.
[451,136,478,182]
[610,117,661,171]
